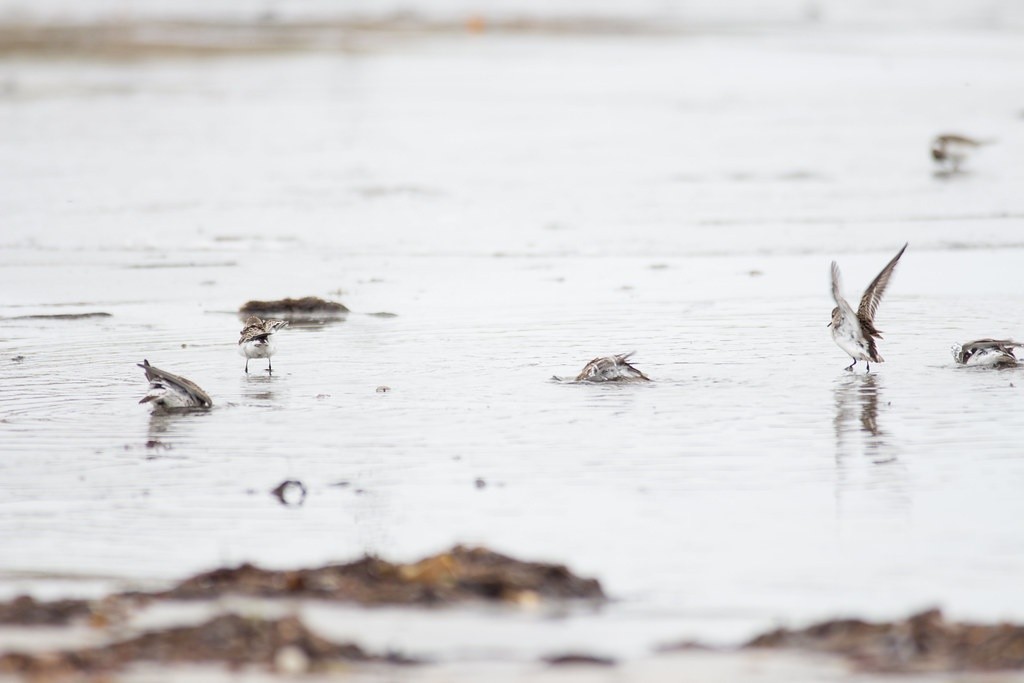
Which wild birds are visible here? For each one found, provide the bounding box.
[825,241,911,373]
[576,349,655,383]
[930,132,1003,178]
[954,337,1023,370]
[237,315,276,373]
[136,358,212,413]
